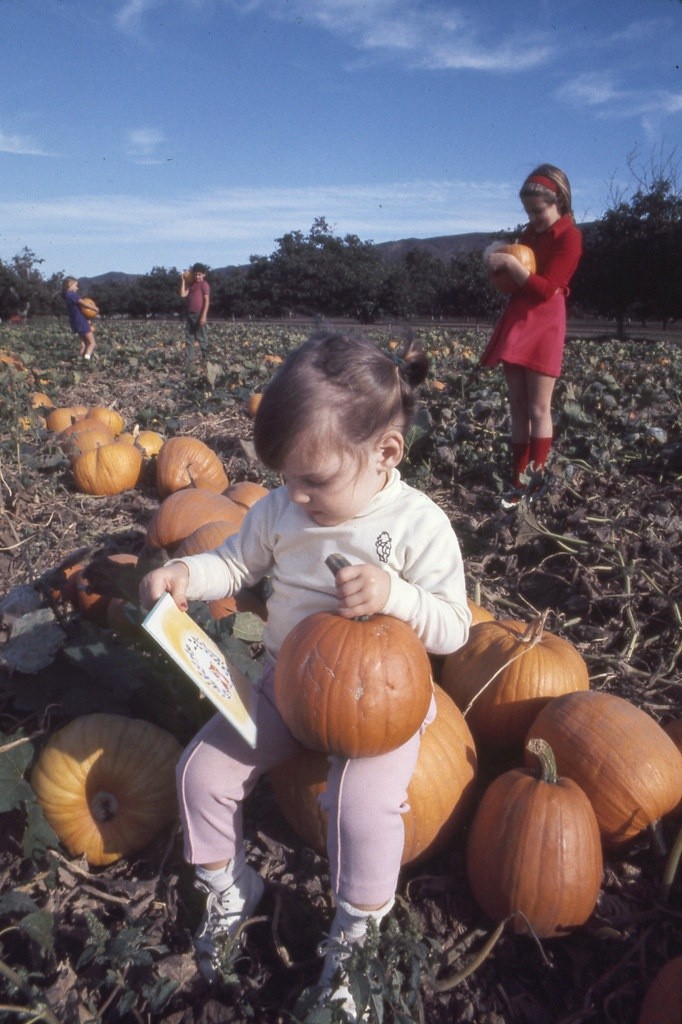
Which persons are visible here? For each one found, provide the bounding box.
[180,263,210,358]
[139,331,475,1024]
[478,164,583,502]
[60,277,99,360]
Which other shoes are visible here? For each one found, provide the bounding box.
[492,484,533,509]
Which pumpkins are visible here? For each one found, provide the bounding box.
[183,270,194,283]
[80,298,98,318]
[0,339,682,1024]
[490,238,536,294]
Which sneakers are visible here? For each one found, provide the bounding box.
[304,914,379,1024]
[191,865,263,985]
